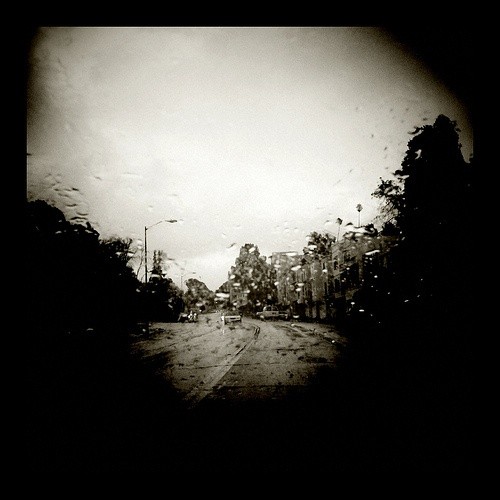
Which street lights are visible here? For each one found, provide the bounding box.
[144,219,178,336]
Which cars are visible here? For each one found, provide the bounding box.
[223,311,241,324]
[256,306,288,320]
